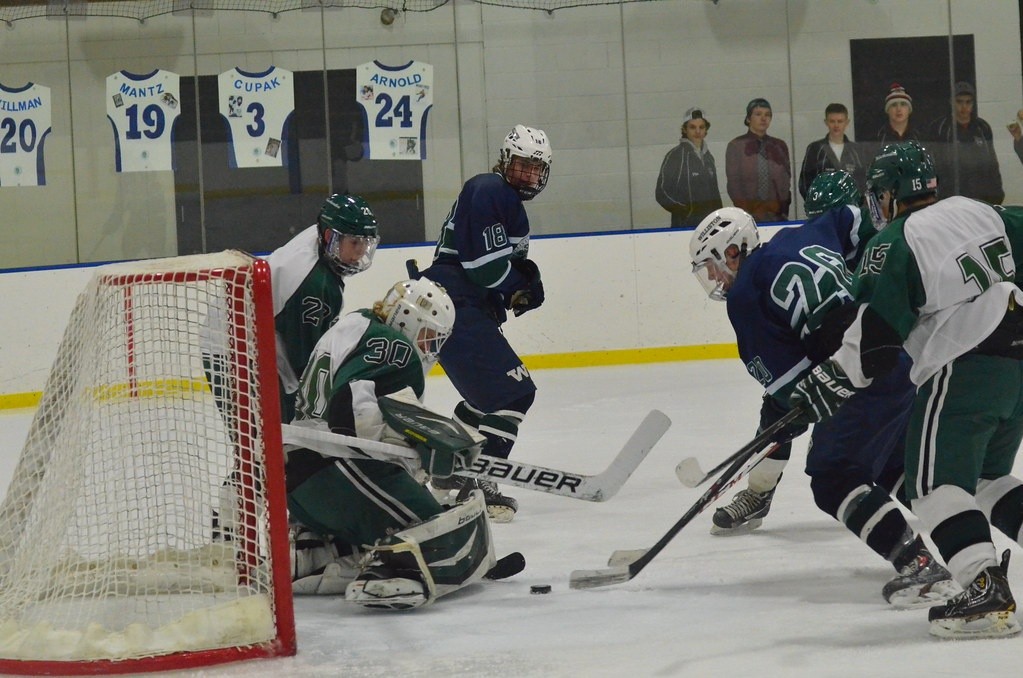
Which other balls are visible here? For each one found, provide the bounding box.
[530,584,552,594]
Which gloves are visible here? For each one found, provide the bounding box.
[484,259,545,317]
[787,359,859,426]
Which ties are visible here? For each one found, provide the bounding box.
[757,139,770,201]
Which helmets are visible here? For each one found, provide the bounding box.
[864,142,939,232]
[689,207,761,301]
[374,276,456,377]
[804,171,865,221]
[318,194,379,278]
[493,125,552,201]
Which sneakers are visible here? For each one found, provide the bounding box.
[880,531,965,607]
[708,471,783,537]
[927,547,1021,637]
[430,426,519,521]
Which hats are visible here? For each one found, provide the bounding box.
[883,83,914,114]
[682,105,711,125]
[951,80,975,99]
[744,98,772,126]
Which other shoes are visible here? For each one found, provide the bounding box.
[345,574,428,611]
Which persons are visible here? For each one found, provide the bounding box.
[421,124,553,522]
[792,143,1023,637]
[653,82,1023,537]
[199,195,498,611]
[690,206,962,610]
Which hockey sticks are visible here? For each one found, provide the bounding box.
[404,258,531,306]
[569,440,779,591]
[672,407,804,491]
[275,403,674,502]
[482,551,527,580]
